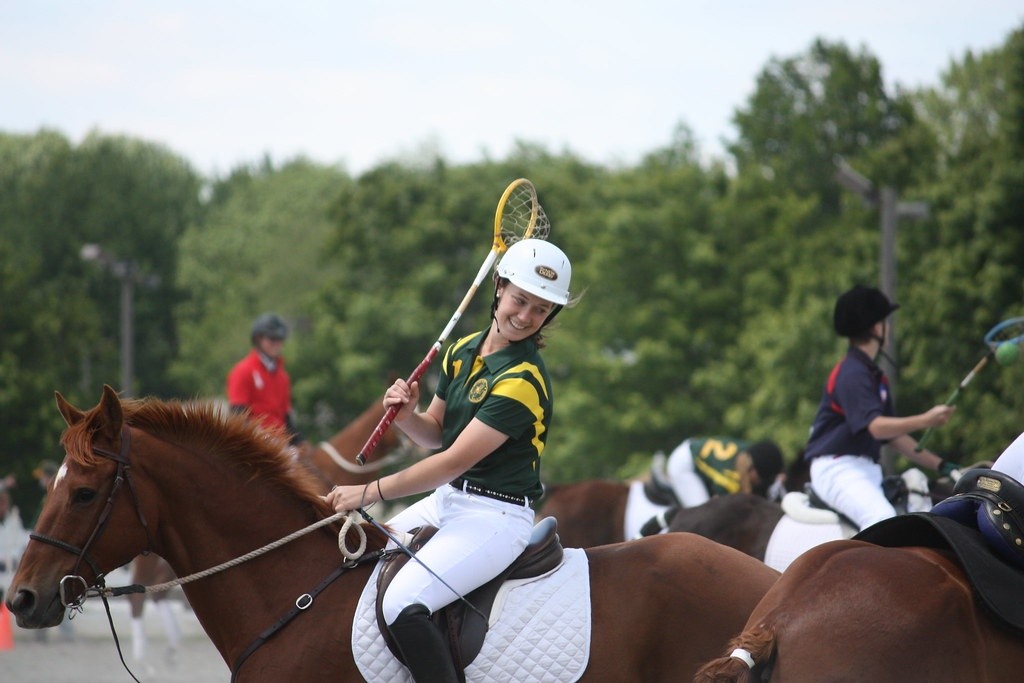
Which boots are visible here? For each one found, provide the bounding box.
[386,604,458,682]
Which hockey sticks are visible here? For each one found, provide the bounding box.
[915,310,1023,455]
[355,175,553,468]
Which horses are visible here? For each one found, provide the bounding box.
[4,386,1020,683]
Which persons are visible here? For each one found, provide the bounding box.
[620,435,784,547]
[225,313,297,448]
[805,284,964,532]
[327,238,572,683]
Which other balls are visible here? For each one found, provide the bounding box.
[994,343,1020,366]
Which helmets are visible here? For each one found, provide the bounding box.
[249,313,288,348]
[496,239,572,306]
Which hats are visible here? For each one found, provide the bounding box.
[747,439,784,496]
[835,284,902,335]
